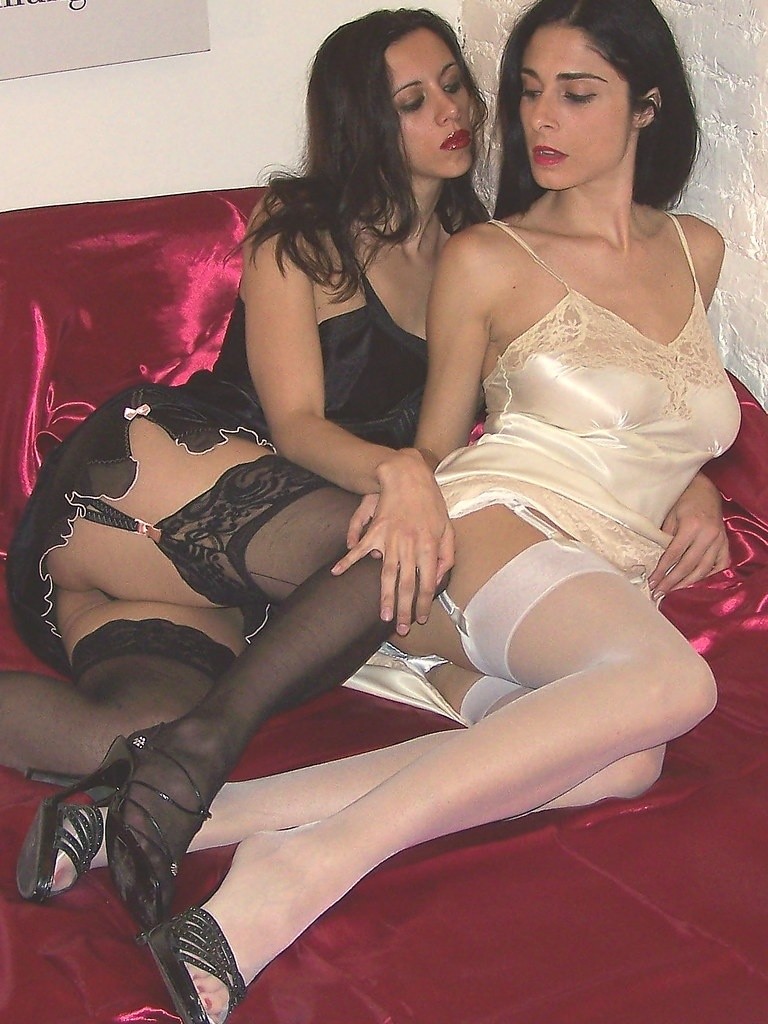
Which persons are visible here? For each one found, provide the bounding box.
[0,8,492,930]
[12,5,740,1024]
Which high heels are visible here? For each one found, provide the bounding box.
[47,722,212,932]
[16,767,117,905]
[133,870,271,1024]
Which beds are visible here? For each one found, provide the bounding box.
[0,188,768,1024]
